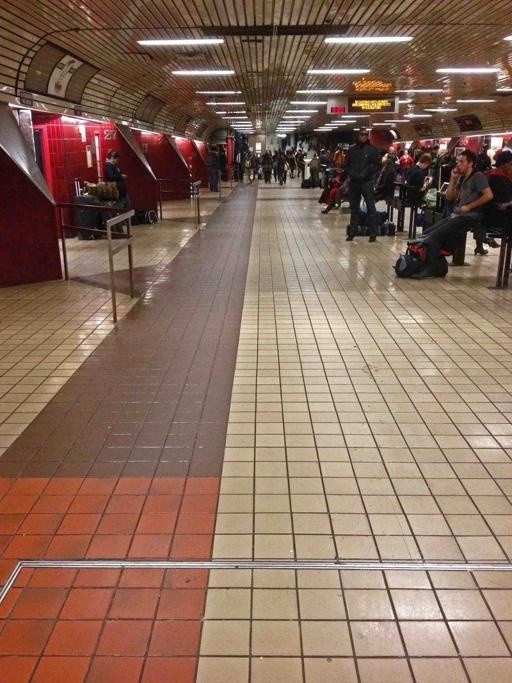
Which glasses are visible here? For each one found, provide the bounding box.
[112,154,118,158]
[360,133,369,136]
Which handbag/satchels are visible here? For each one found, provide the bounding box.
[127,209,158,224]
[347,211,396,237]
[391,234,450,279]
[83,181,120,201]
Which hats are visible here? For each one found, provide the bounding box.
[106,149,118,158]
[492,150,512,167]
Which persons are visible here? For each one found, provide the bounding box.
[105,150,129,225]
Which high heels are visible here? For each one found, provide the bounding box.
[474,246,488,256]
[488,238,502,248]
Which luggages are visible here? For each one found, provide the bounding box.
[73,177,102,240]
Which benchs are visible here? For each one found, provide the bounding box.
[385,179,511,298]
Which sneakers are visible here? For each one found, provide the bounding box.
[346,230,356,241]
[368,230,377,243]
